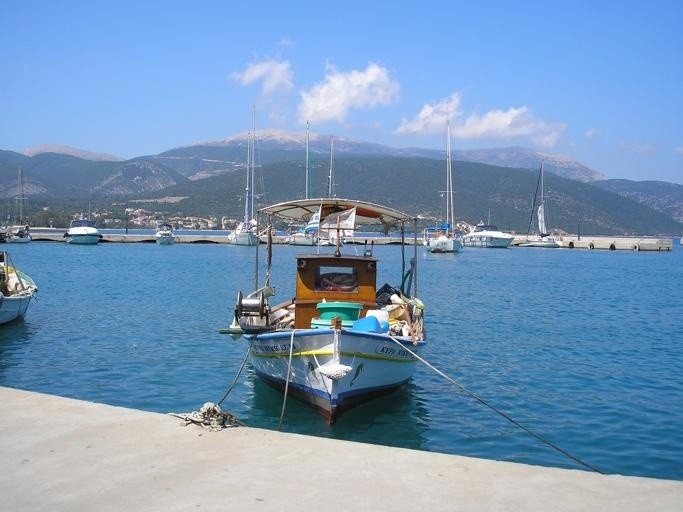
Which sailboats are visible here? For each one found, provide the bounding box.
[424,126,461,252]
[227,122,344,245]
[518,160,561,247]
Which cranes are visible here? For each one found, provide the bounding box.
[13,166,28,225]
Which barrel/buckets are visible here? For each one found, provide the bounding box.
[316,302,362,320]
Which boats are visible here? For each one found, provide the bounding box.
[219,199,427,425]
[462,225,514,247]
[1,250,36,324]
[0,225,30,243]
[156,224,174,244]
[63,221,103,244]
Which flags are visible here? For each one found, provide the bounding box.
[304,206,319,231]
